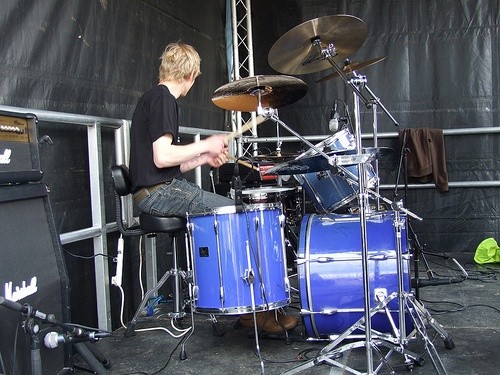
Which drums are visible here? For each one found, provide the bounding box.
[186,202,292,316]
[242,186,299,224]
[259,164,278,186]
[289,128,380,212]
[297,212,417,338]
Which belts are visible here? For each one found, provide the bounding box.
[135,185,158,204]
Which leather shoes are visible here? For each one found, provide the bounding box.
[240,312,298,334]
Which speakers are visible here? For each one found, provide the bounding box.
[0,181,76,375]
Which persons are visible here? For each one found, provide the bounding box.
[129,43,298,335]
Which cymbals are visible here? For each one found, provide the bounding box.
[268,14,368,75]
[210,74,309,112]
[252,153,300,158]
[314,56,387,84]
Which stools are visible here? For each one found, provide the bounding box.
[110,164,224,361]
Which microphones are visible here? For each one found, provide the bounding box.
[330,100,338,131]
[411,278,465,288]
[44,333,111,348]
[232,162,240,189]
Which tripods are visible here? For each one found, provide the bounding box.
[257,55,457,375]
